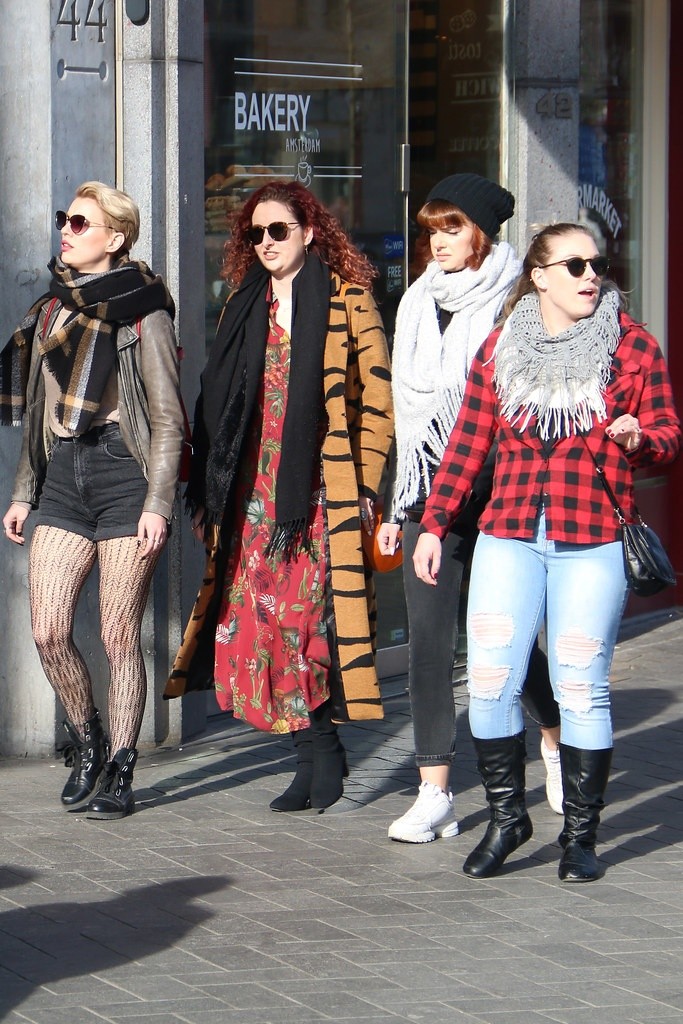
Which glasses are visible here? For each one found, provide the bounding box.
[55,210,118,234]
[530,256,609,281]
[246,221,305,245]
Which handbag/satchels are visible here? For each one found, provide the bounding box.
[361,492,403,573]
[622,523,677,597]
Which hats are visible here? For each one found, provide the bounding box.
[426,173,515,239]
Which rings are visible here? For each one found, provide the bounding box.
[360,507,368,520]
[3,530,6,534]
[147,538,156,542]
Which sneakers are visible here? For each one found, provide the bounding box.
[541,737,564,814]
[388,781,459,843]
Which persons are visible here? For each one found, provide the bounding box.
[379,169,564,845]
[162,179,396,813]
[2,177,191,823]
[409,222,683,883]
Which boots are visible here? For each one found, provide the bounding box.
[270,727,313,812]
[556,741,614,881]
[61,707,111,810]
[86,743,139,819]
[463,727,533,879]
[308,699,350,809]
